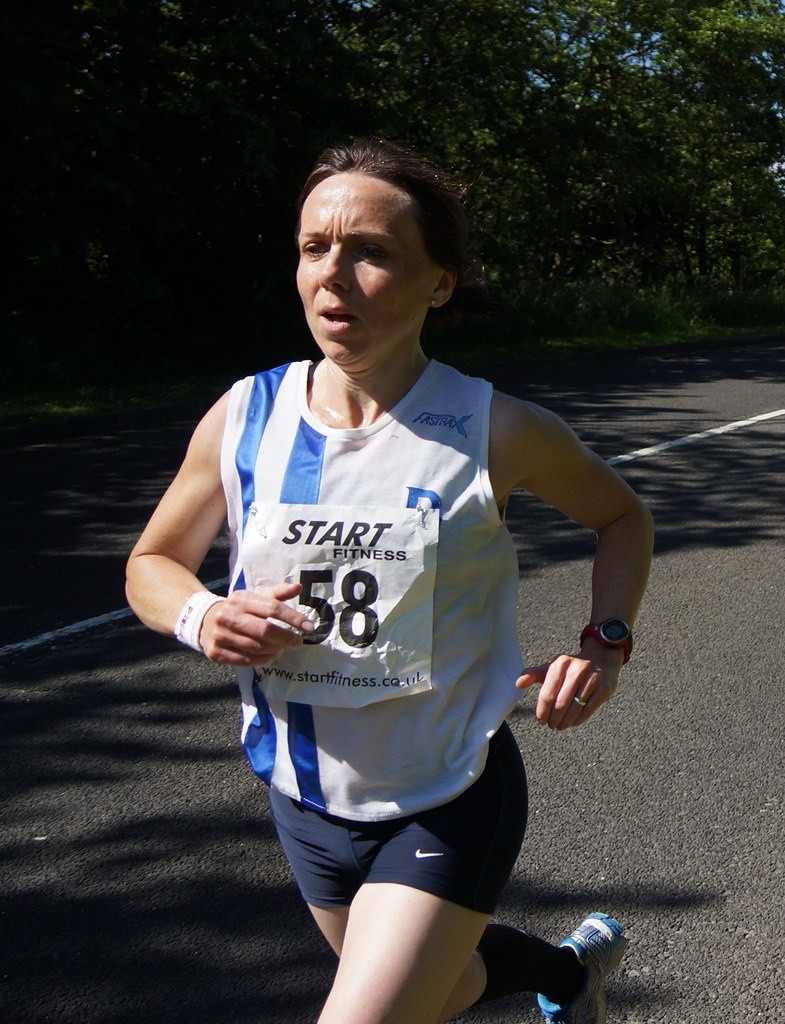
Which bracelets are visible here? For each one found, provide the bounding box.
[174,593,226,652]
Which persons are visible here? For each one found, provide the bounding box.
[121,139,656,1023]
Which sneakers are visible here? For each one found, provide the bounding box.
[537,912,631,1024]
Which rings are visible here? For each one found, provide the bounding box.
[574,696,586,706]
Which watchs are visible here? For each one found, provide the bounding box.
[579,618,633,665]
